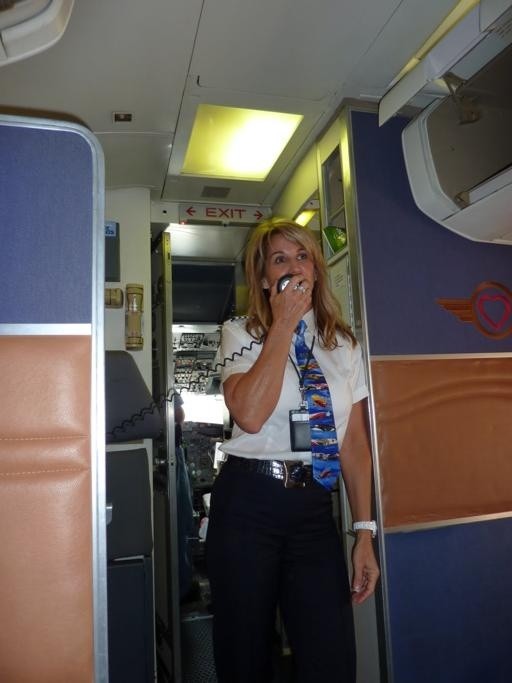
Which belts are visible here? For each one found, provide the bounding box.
[257,459,313,489]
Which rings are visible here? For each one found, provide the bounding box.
[297,286,306,293]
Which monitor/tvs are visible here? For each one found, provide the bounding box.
[172,264,235,321]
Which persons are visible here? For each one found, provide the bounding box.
[205,218,380,683]
[174,387,201,606]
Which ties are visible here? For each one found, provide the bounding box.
[295,318,341,492]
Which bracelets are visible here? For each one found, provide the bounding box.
[353,520,378,538]
